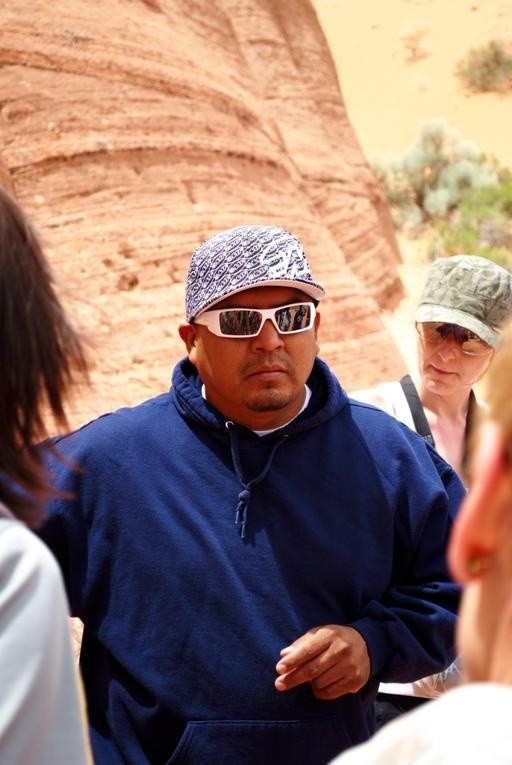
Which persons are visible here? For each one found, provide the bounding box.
[340,249,512,734]
[0,191,101,765]
[0,225,469,764]
[329,320,512,763]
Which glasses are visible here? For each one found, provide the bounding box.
[417,322,494,358]
[193,302,317,339]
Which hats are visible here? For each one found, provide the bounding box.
[415,253,512,349]
[184,223,325,324]
